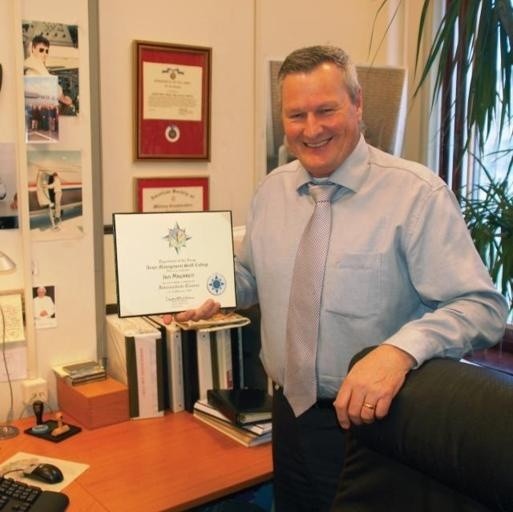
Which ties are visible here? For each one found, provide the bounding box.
[282,180,341,419]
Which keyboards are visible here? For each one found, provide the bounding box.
[0,476,68,512]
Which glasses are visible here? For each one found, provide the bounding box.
[38,47,49,54]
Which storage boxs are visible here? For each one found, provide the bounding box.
[57,375,129,430]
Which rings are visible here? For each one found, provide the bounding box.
[362,403,374,409]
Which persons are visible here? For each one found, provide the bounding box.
[41,172,62,222]
[24,35,72,105]
[32,287,56,320]
[163,44,509,512]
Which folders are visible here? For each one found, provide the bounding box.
[205,386,283,428]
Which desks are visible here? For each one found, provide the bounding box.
[0,410,273,512]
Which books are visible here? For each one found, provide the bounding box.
[192,388,275,448]
[50,361,106,387]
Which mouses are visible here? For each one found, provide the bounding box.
[23,464,64,484]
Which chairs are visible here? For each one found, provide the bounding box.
[334,343,509,511]
